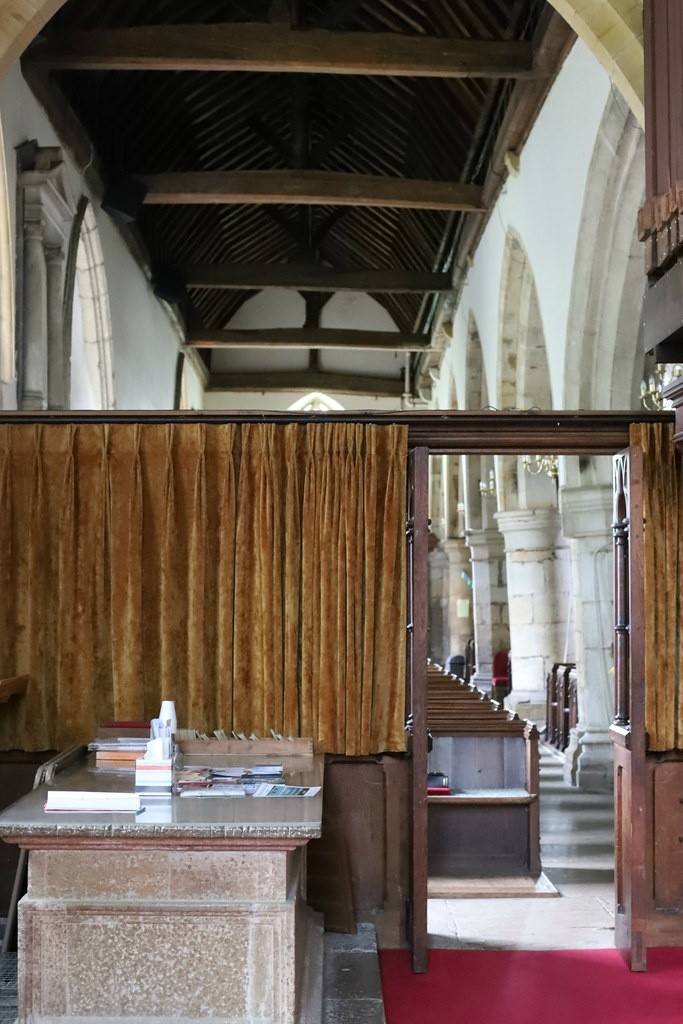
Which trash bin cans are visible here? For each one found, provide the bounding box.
[445,655,464,679]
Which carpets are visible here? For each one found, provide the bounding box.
[378,945,683,1024]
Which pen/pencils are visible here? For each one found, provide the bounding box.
[133,807,145,815]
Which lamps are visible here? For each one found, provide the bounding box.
[523,454,558,484]
[638,362,683,412]
[478,469,495,496]
[457,502,465,516]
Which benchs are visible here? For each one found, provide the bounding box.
[427,657,542,876]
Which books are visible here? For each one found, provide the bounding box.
[42,737,153,813]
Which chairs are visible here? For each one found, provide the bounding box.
[489,651,510,702]
